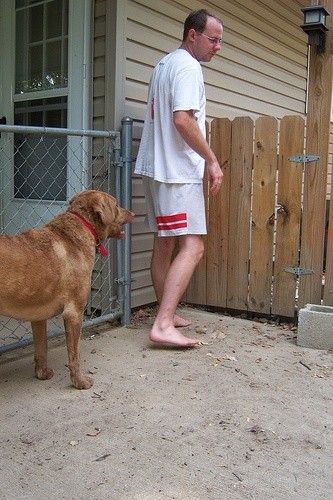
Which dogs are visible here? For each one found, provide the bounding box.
[0,189,136,390]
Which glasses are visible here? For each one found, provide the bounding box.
[196,30,224,46]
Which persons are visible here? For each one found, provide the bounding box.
[133,10,224,346]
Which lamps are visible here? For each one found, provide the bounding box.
[300,4,330,54]
